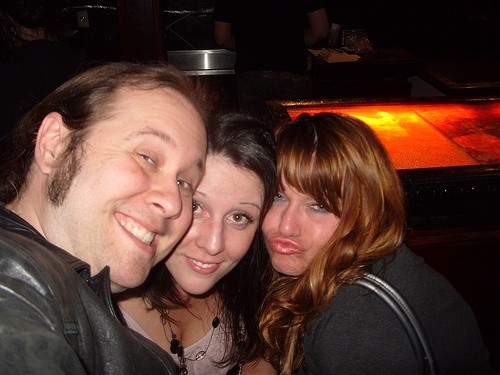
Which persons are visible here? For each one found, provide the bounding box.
[0,60,220,375]
[109,111,277,375]
[0,0,95,140]
[258,109,496,374]
[213,0,330,76]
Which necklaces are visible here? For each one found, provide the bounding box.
[151,296,221,375]
